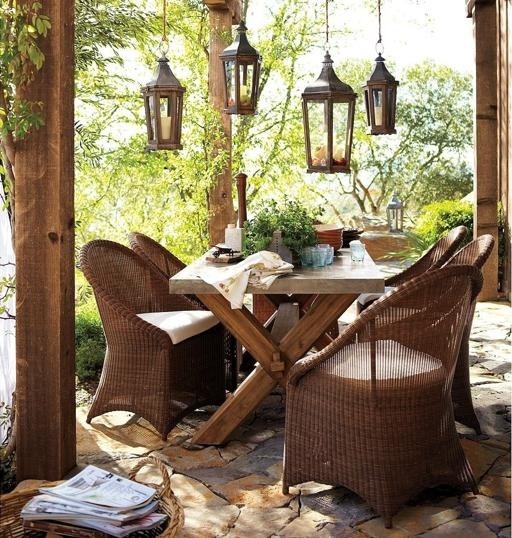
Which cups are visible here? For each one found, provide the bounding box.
[350,241,366,262]
[300,244,334,267]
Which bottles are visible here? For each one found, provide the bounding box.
[224,224,241,255]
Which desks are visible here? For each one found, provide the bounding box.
[169,240,384,446]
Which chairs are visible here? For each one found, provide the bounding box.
[79,239,231,445]
[440,233,495,435]
[357,226,466,314]
[283,263,486,526]
[126,230,244,394]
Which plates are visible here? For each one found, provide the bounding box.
[306,225,364,257]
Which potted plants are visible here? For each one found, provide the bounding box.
[243,194,326,269]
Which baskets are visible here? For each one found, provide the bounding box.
[0,458,185,538]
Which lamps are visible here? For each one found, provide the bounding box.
[299,0,358,174]
[219,0,259,115]
[140,0,186,152]
[361,0,399,136]
[386,187,404,234]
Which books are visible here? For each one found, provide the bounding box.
[20,462,168,537]
